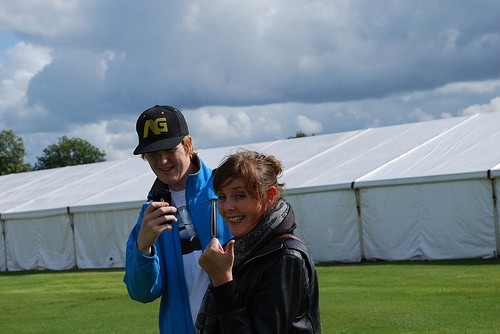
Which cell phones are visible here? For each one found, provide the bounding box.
[153,190,173,225]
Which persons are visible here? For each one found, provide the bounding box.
[196,148,321,334]
[123,105,235,334]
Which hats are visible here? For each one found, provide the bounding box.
[133,105,189,155]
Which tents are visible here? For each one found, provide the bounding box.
[0,113,500,272]
